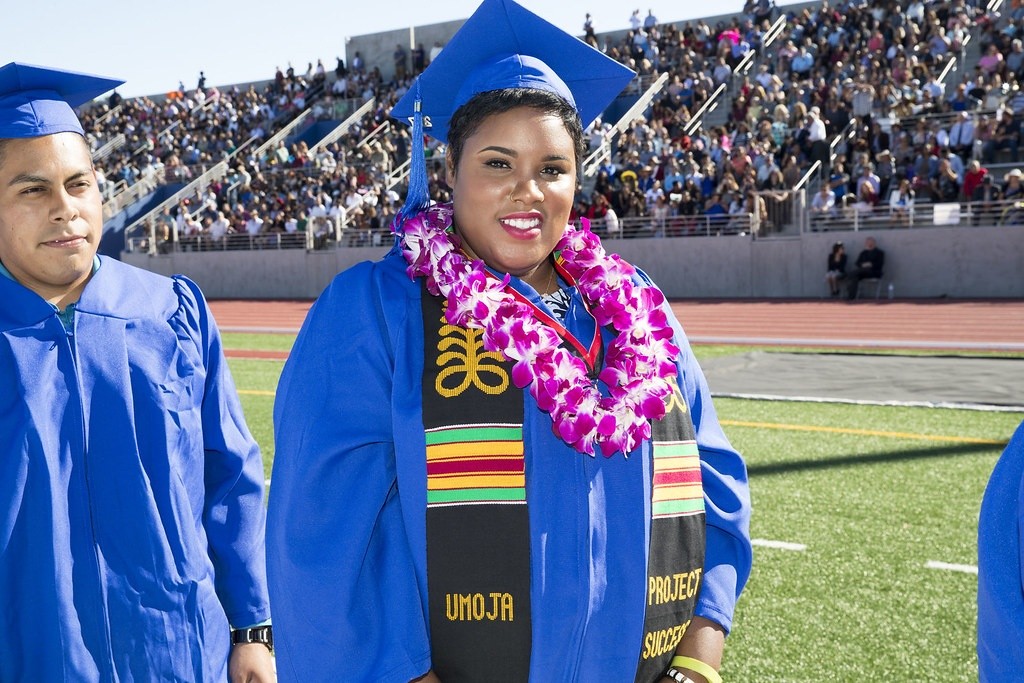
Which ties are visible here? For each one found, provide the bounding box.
[957,122,964,144]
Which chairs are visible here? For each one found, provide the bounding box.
[863,269,888,301]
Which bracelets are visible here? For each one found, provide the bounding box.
[667,652,726,683]
[229,619,277,652]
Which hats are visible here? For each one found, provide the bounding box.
[841,78,855,87]
[917,117,927,125]
[388,0,638,144]
[891,123,902,130]
[1004,169,1024,182]
[931,120,940,127]
[1,60,125,134]
[620,170,637,182]
[875,149,890,161]
[639,165,653,176]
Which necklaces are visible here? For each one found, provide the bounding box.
[399,204,679,458]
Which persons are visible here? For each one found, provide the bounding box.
[847,236,887,302]
[264,0,758,683]
[572,1,1024,232]
[824,240,846,296]
[70,42,450,251]
[0,63,277,683]
[973,417,1024,683]
